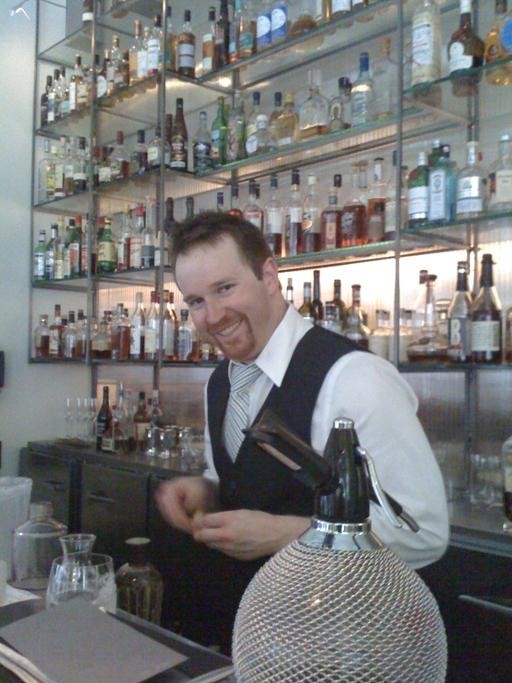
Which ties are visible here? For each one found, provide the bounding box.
[224,364,262,463]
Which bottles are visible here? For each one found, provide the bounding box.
[161,290,221,362]
[39,135,88,204]
[505,306,512,363]
[302,173,324,253]
[34,304,87,360]
[94,195,159,272]
[350,51,372,128]
[339,161,369,258]
[171,98,188,172]
[428,144,457,227]
[332,174,343,207]
[225,90,246,163]
[163,197,175,267]
[327,77,350,133]
[320,186,342,251]
[455,140,485,218]
[402,38,424,116]
[295,68,331,142]
[40,54,90,133]
[486,135,512,213]
[448,261,473,362]
[470,254,502,364]
[485,0,512,86]
[176,9,197,81]
[243,179,262,235]
[427,139,442,167]
[246,92,263,141]
[112,0,128,18]
[90,291,160,360]
[263,174,283,258]
[447,1,483,98]
[356,160,372,209]
[411,0,443,107]
[285,169,303,257]
[93,113,172,192]
[406,151,430,230]
[115,538,163,627]
[143,26,151,50]
[186,196,195,221]
[12,501,67,591]
[269,92,283,135]
[383,149,408,240]
[193,111,210,177]
[228,0,392,72]
[216,192,223,213]
[201,6,218,83]
[502,436,512,523]
[286,270,450,364]
[367,157,386,243]
[33,212,90,281]
[272,92,299,148]
[94,35,134,107]
[309,36,311,38]
[148,15,161,84]
[96,381,163,456]
[79,0,93,24]
[228,185,243,218]
[158,6,176,81]
[245,114,278,157]
[371,37,400,120]
[129,20,146,86]
[212,0,232,77]
[210,96,226,168]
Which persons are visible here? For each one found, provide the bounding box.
[152,210,452,615]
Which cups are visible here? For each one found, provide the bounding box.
[158,429,175,460]
[178,429,193,458]
[45,553,116,617]
[470,453,503,508]
[144,428,157,458]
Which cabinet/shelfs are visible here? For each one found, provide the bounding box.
[28,1,512,373]
[19,434,512,683]
